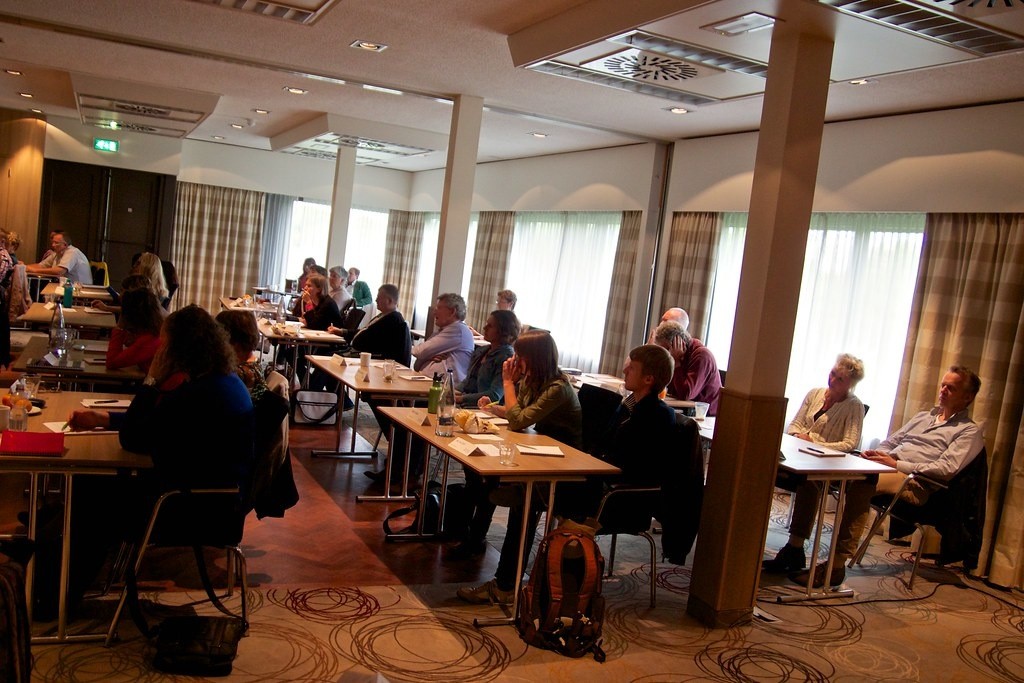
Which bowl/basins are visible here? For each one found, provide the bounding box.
[51,327,76,350]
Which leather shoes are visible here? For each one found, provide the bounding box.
[762,545,806,568]
[789,562,845,588]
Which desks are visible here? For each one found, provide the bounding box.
[218,297,293,367]
[565,373,697,416]
[304,355,462,501]
[683,415,897,603]
[256,320,346,393]
[410,328,492,346]
[10,271,64,330]
[11,336,147,393]
[40,282,113,307]
[21,302,117,339]
[251,284,302,309]
[0,387,153,645]
[377,406,620,627]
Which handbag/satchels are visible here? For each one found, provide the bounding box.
[292,389,339,426]
[151,616,249,676]
[382,480,469,540]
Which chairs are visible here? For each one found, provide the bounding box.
[0,255,990,649]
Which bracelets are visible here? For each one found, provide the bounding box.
[142,375,158,384]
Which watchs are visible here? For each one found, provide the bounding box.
[276,259,372,385]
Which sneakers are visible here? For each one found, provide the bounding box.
[456,579,515,605]
[490,488,522,507]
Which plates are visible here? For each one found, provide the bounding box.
[26,398,46,414]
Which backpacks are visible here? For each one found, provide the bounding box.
[515,522,612,663]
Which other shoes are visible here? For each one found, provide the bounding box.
[364,468,424,492]
[448,540,489,555]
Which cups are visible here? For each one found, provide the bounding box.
[695,402,710,421]
[499,440,516,466]
[72,344,85,368]
[383,359,395,381]
[0,374,41,432]
[658,387,667,401]
[359,352,371,367]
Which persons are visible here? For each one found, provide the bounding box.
[497,291,516,312]
[132,252,170,305]
[442,310,520,409]
[446,328,584,557]
[106,287,175,381]
[215,310,270,406]
[0,227,21,288]
[308,284,405,412]
[762,365,984,588]
[786,356,865,453]
[18,229,93,285]
[645,307,723,417]
[363,293,475,486]
[456,343,677,603]
[0,302,254,621]
[91,273,170,320]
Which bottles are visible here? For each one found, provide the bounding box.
[435,368,455,436]
[277,297,286,324]
[8,384,28,432]
[428,371,443,414]
[63,278,73,308]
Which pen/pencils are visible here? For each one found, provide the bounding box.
[60,414,78,432]
[95,400,118,403]
[93,359,107,361]
[515,442,538,450]
[479,401,499,409]
[807,446,825,454]
[411,377,426,379]
[331,323,333,329]
